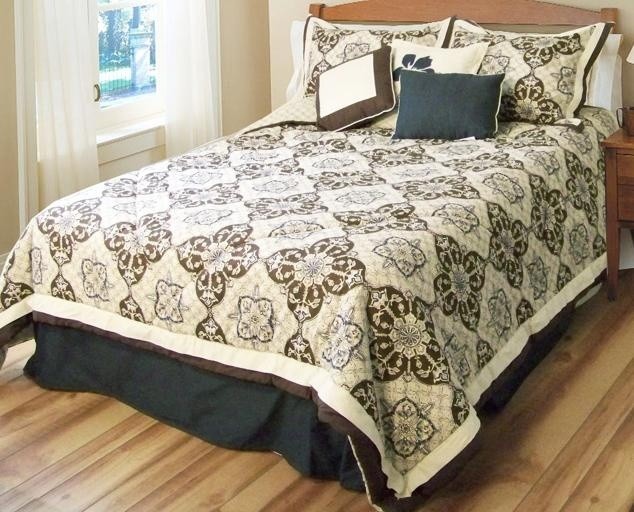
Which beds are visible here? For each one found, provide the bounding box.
[0,0,623,512]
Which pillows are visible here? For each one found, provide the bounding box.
[447,16,616,133]
[312,44,397,135]
[371,38,491,129]
[390,68,506,141]
[299,16,456,101]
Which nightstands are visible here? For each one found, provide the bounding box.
[601,128,634,300]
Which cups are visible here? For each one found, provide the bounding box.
[615,105,634,137]
